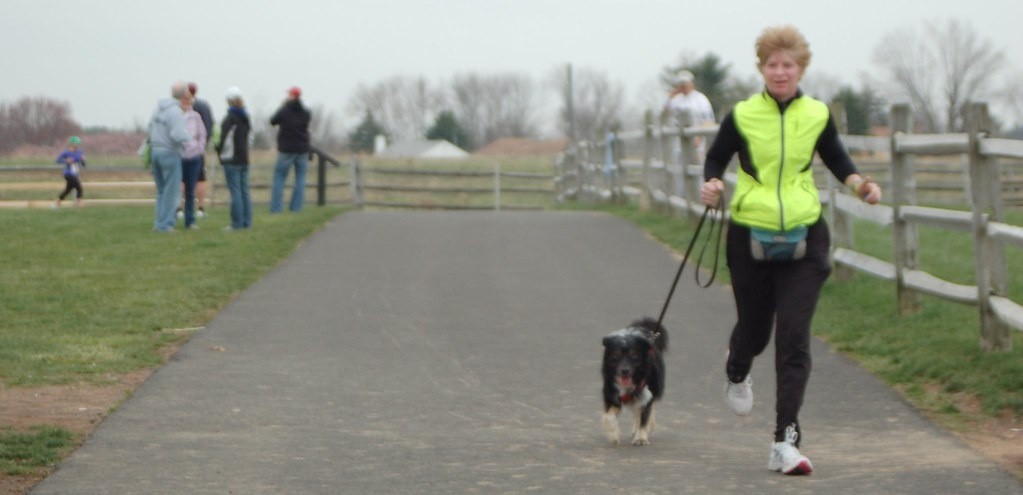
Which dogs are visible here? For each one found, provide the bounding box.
[600,317,670,448]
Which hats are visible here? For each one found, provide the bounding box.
[69,136,81,144]
[223,87,240,100]
[669,70,695,88]
[285,85,302,97]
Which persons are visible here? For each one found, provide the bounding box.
[53,136,87,208]
[176,83,213,217]
[143,82,193,232]
[214,87,253,231]
[667,70,716,220]
[701,25,881,477]
[179,92,207,229]
[269,87,313,214]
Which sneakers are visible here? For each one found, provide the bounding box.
[188,223,199,229]
[195,207,206,218]
[49,203,58,209]
[219,225,233,231]
[723,348,754,414]
[176,208,184,218]
[767,422,814,476]
[153,222,183,233]
